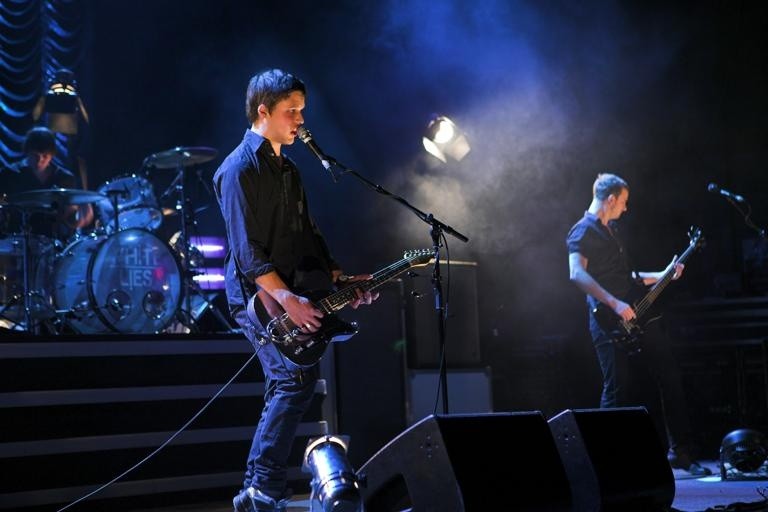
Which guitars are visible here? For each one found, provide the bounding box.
[247,248,439,366]
[592,225,705,356]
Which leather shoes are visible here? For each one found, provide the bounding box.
[667,448,711,475]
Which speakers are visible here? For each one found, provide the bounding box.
[353,410,574,511]
[549,407,676,511]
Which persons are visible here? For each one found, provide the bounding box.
[566,172,713,477]
[2,125,77,318]
[33,67,88,187]
[212,66,382,512]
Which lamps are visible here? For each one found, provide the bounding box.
[718,428,767,482]
[299,421,371,512]
[418,110,472,167]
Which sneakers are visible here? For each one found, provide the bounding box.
[232,487,289,512]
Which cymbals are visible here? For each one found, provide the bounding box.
[143,147,217,169]
[4,189,105,209]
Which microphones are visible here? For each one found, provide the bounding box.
[293,126,333,173]
[706,181,744,206]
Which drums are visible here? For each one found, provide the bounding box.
[0,232,66,321]
[96,173,164,235]
[49,227,185,335]
